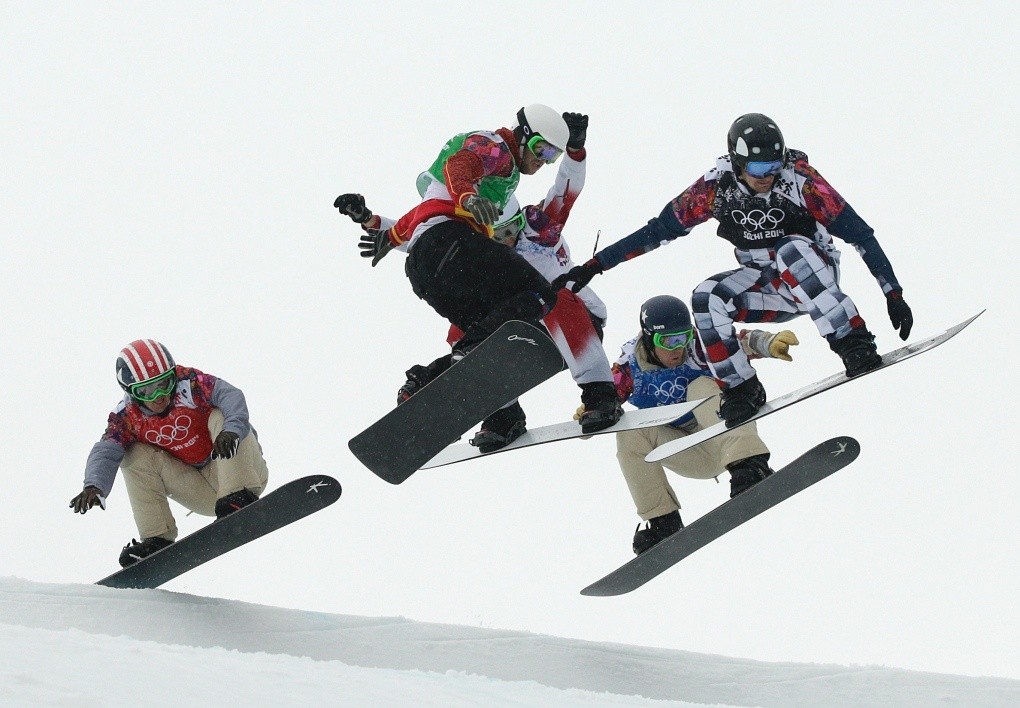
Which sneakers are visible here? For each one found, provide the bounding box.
[119,537,174,567]
[632,511,685,555]
[215,489,259,519]
[730,457,772,498]
[832,330,883,378]
[397,351,767,453]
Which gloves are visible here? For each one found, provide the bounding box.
[210,432,240,460]
[465,195,499,226]
[573,404,585,421]
[563,112,588,148]
[333,194,372,223]
[358,229,395,267]
[887,291,914,341]
[769,331,799,362]
[70,487,107,515]
[552,257,602,293]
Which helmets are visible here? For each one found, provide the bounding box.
[493,193,520,225]
[116,340,176,394]
[728,114,785,176]
[640,295,693,341]
[516,105,570,150]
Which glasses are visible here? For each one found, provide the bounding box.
[491,214,526,243]
[132,370,177,402]
[747,162,785,178]
[528,136,563,164]
[653,329,693,351]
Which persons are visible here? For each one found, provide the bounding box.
[333,103,624,455]
[573,295,799,556]
[69,338,269,568]
[550,113,914,428]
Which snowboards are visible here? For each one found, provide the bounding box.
[418,394,717,470]
[348,320,565,485]
[643,308,987,463]
[93,474,342,590]
[580,436,860,597]
